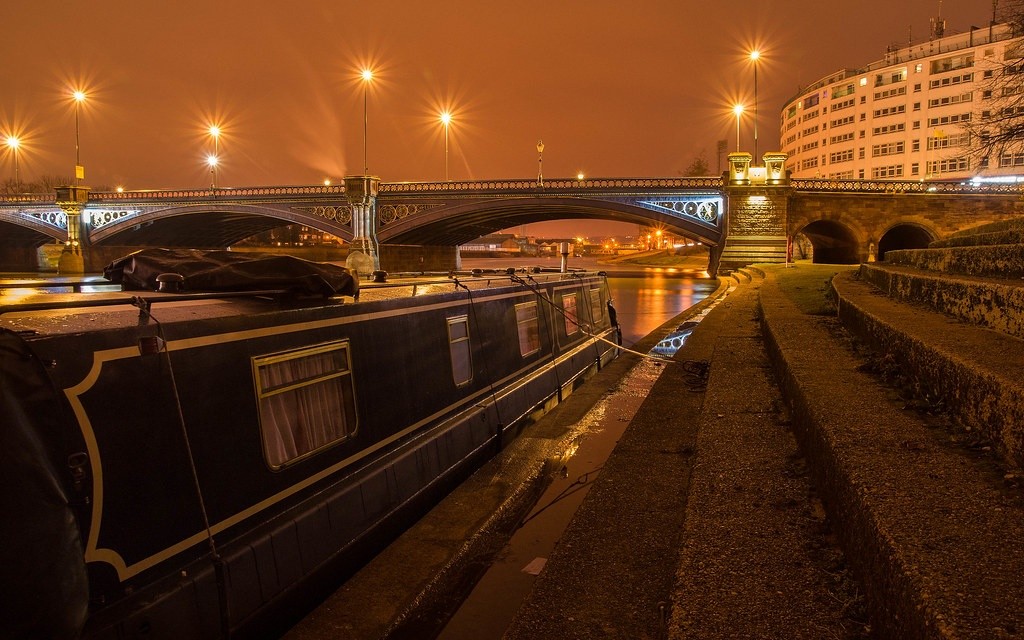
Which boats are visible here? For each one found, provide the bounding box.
[0,242,622,640]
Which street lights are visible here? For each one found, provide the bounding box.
[751,49,759,166]
[733,103,742,152]
[10,138,18,196]
[363,68,370,175]
[208,123,219,190]
[73,89,81,187]
[443,112,450,181]
